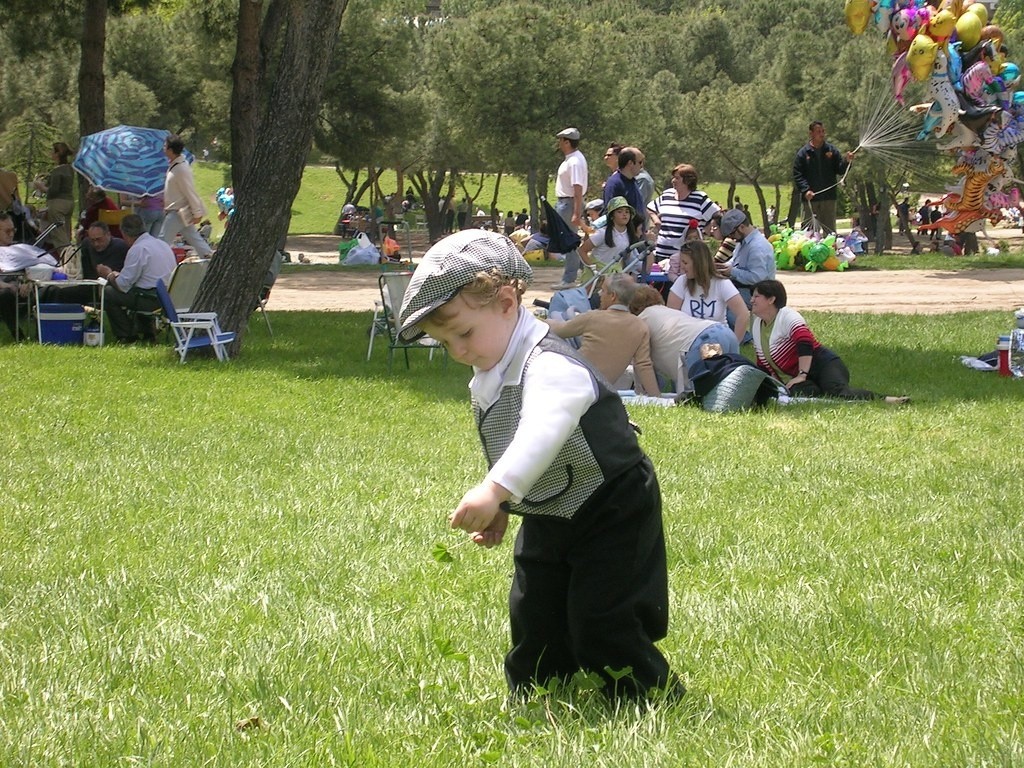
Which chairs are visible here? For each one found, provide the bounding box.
[353,214,427,240]
[367,269,449,372]
[98,209,133,227]
[129,259,271,363]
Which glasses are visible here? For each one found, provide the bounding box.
[5,228,17,235]
[729,228,738,237]
[89,236,104,244]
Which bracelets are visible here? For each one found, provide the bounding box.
[106,273,112,278]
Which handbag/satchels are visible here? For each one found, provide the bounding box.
[339,232,381,265]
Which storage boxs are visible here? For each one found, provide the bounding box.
[33,303,86,347]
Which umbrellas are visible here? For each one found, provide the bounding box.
[71,124,195,214]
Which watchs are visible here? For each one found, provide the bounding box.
[798,369,809,375]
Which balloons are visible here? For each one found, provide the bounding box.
[216,187,235,228]
[768,1,1024,272]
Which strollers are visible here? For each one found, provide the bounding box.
[533,241,656,323]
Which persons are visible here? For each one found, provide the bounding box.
[396,228,687,710]
[0,134,213,344]
[254,252,278,310]
[203,147,209,161]
[340,121,981,407]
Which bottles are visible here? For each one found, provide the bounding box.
[175,233,183,247]
[996,337,1013,376]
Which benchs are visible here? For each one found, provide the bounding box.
[471,215,500,228]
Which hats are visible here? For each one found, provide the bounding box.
[397,229,534,345]
[720,209,746,237]
[557,128,579,140]
[607,196,636,221]
